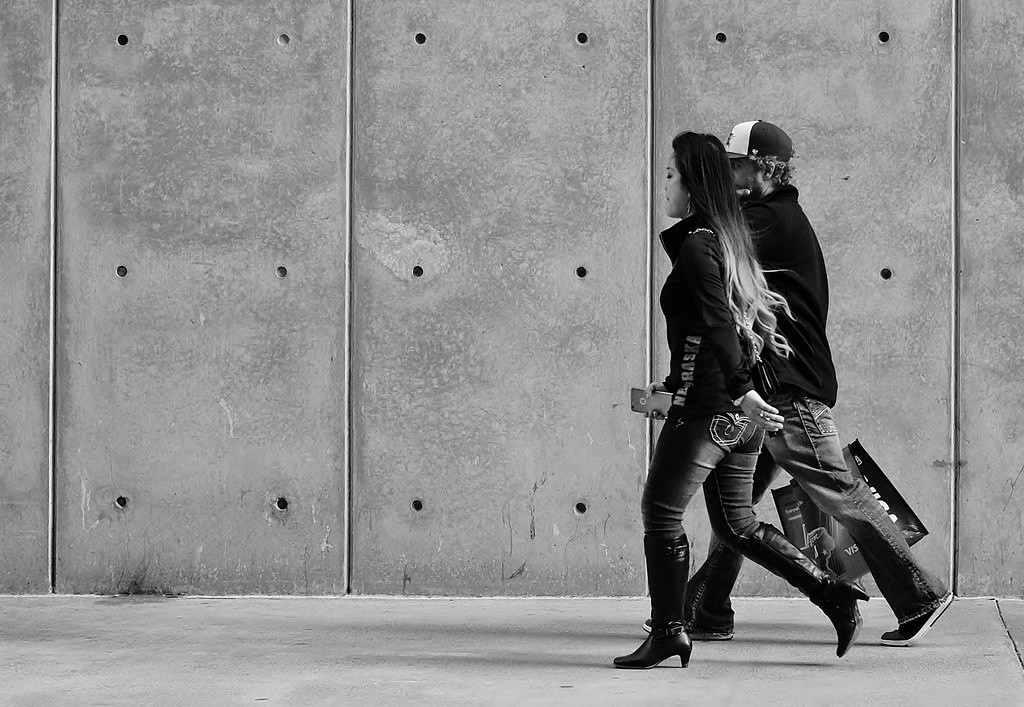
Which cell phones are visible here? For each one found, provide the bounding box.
[630,388,673,417]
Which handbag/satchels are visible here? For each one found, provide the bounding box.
[751,357,781,404]
[770,438,930,585]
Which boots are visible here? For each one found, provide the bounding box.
[737,521,871,659]
[613,533,693,669]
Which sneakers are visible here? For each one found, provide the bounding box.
[880,589,955,646]
[643,619,734,642]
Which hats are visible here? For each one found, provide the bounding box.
[724,120,794,159]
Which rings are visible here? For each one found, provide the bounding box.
[758,410,765,418]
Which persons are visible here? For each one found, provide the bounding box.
[610,132,870,669]
[643,120,956,644]
